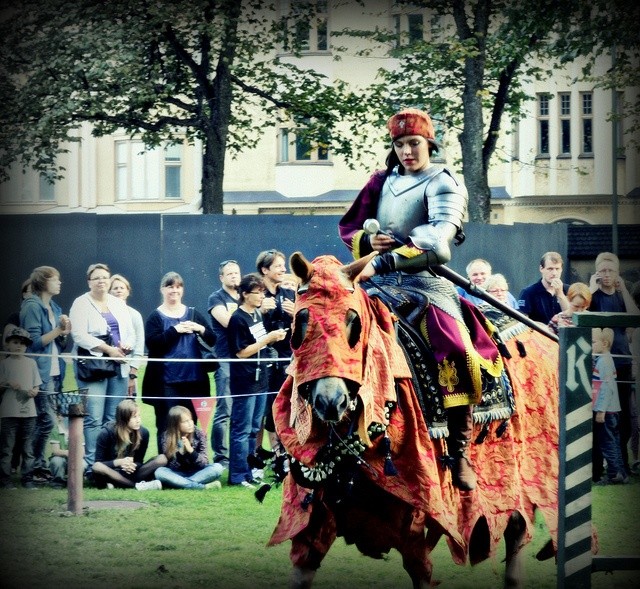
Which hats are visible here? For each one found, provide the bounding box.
[388,108,439,154]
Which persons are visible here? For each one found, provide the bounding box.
[19,266,72,483]
[69,263,136,485]
[1,323,49,491]
[154,405,223,490]
[339,108,505,491]
[518,251,573,325]
[207,261,247,463]
[21,279,33,300]
[466,259,519,314]
[227,276,290,491]
[247,249,295,459]
[92,399,168,489]
[590,252,640,479]
[548,282,592,333]
[109,273,146,400]
[592,328,622,483]
[141,271,218,456]
[487,273,508,302]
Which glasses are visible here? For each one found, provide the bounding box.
[89,276,109,282]
[250,290,267,296]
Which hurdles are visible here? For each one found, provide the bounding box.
[557,307,640,584]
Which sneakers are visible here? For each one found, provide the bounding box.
[596,471,624,485]
[107,482,115,489]
[206,480,222,490]
[249,479,267,486]
[238,481,256,489]
[135,480,162,492]
[22,479,38,490]
[7,483,18,490]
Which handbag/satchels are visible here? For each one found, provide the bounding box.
[189,305,220,373]
[77,335,117,383]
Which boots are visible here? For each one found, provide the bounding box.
[447,403,477,491]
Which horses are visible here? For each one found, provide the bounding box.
[266,248,559,588]
